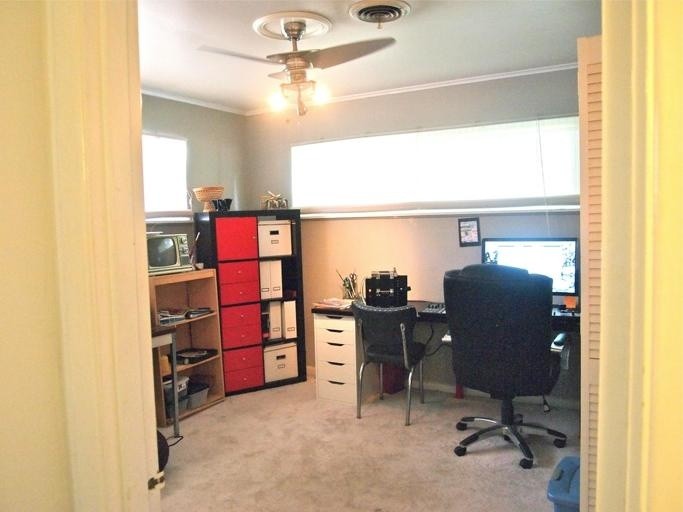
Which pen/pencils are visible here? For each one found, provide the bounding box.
[335,269,355,299]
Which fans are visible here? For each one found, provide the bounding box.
[194,11,395,121]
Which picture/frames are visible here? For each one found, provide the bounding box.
[458,218,480,247]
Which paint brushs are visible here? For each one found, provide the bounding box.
[190,232,200,258]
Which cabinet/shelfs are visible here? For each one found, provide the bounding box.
[149,268,225,427]
[313,309,380,406]
[194,209,307,396]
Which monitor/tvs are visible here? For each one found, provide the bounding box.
[146,233,193,277]
[481,237,579,296]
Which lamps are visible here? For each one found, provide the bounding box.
[279,71,317,119]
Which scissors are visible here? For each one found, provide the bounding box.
[350,273,357,282]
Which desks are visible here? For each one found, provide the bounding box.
[151,324,183,446]
[406,299,580,330]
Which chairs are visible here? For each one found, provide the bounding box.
[443,265,568,470]
[353,300,428,426]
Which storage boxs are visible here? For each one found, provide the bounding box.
[546,456,580,512]
[263,341,299,384]
[257,220,293,257]
